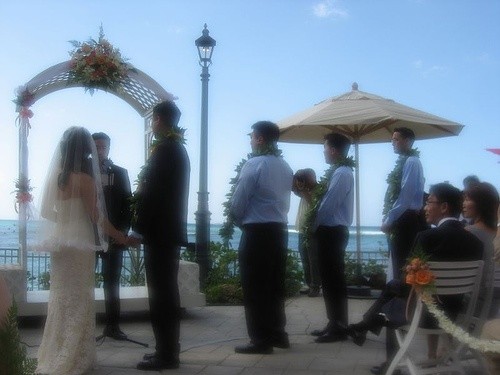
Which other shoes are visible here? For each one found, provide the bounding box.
[235,341,273,354]
[334,320,366,346]
[104,326,127,340]
[370,363,401,375]
[272,336,289,349]
[314,330,349,343]
[137,357,179,371]
[311,327,329,336]
[143,353,157,359]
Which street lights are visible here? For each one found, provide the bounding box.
[194,22,216,281]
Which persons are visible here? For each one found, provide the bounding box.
[462,182,500,305]
[92,132,132,338]
[127,101,190,370]
[310,134,355,343]
[292,169,322,297]
[345,183,485,375]
[382,127,425,278]
[35,125,130,375]
[231,120,293,354]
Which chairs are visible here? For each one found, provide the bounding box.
[454,260,500,375]
[385,260,485,375]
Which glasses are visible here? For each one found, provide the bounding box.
[425,199,442,205]
[391,137,404,141]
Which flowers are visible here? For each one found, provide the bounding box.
[13,172,37,214]
[405,248,500,355]
[129,125,188,227]
[13,85,38,136]
[302,155,356,250]
[379,146,422,256]
[219,138,284,249]
[66,20,137,98]
[290,168,319,204]
